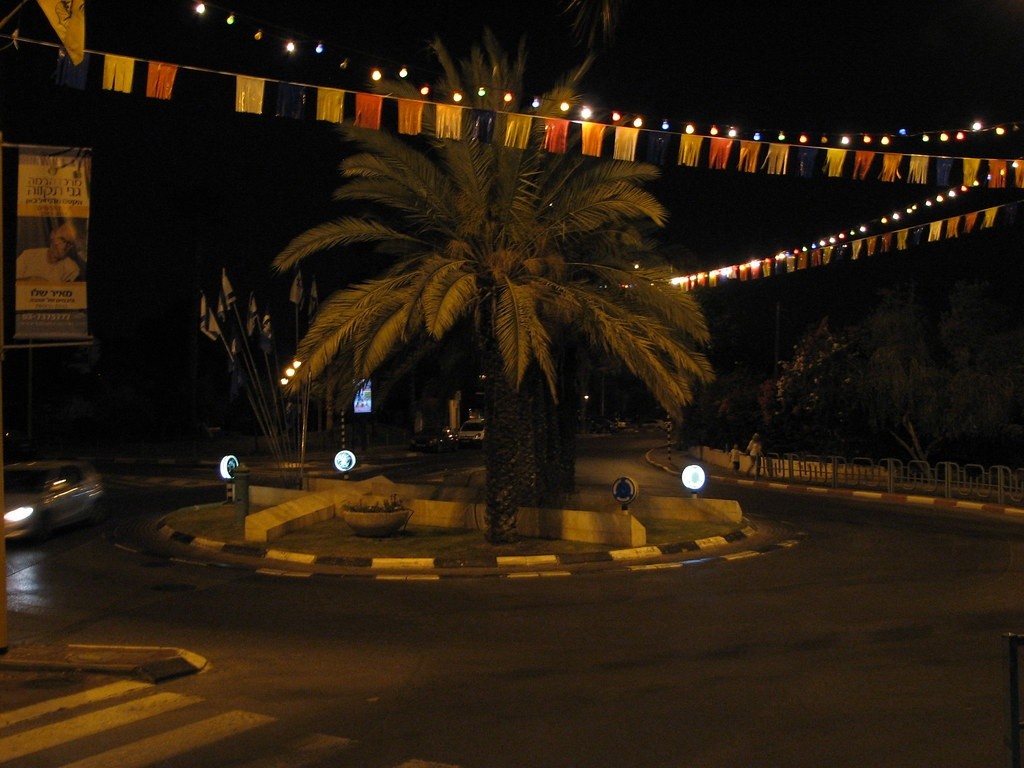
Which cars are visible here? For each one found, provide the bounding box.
[642,420,664,431]
[455,418,485,443]
[4,459,108,541]
[411,425,456,455]
[586,417,627,432]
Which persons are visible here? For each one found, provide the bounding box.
[745,433,762,480]
[728,444,745,476]
